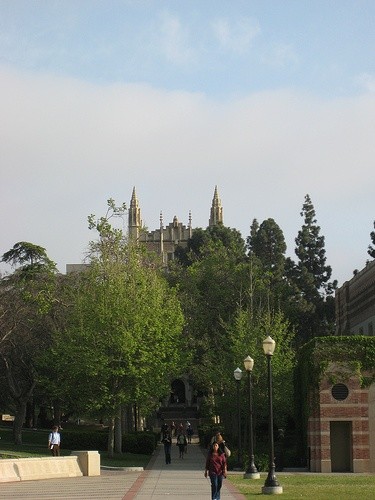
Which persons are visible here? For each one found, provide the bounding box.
[211,431,231,457]
[205,441,227,500]
[48,426,60,456]
[161,420,192,464]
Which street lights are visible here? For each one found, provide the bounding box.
[243,355,261,480]
[261,336,282,495]
[233,366,243,471]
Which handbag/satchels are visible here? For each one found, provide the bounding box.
[223,465,227,478]
[224,446,230,456]
[185,447,188,454]
